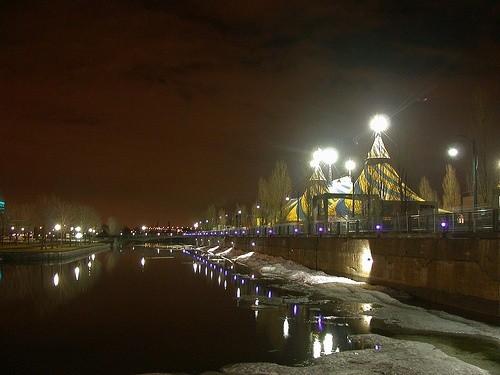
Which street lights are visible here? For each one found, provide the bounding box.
[346,159,355,218]
[286,189,300,231]
[448,134,478,232]
